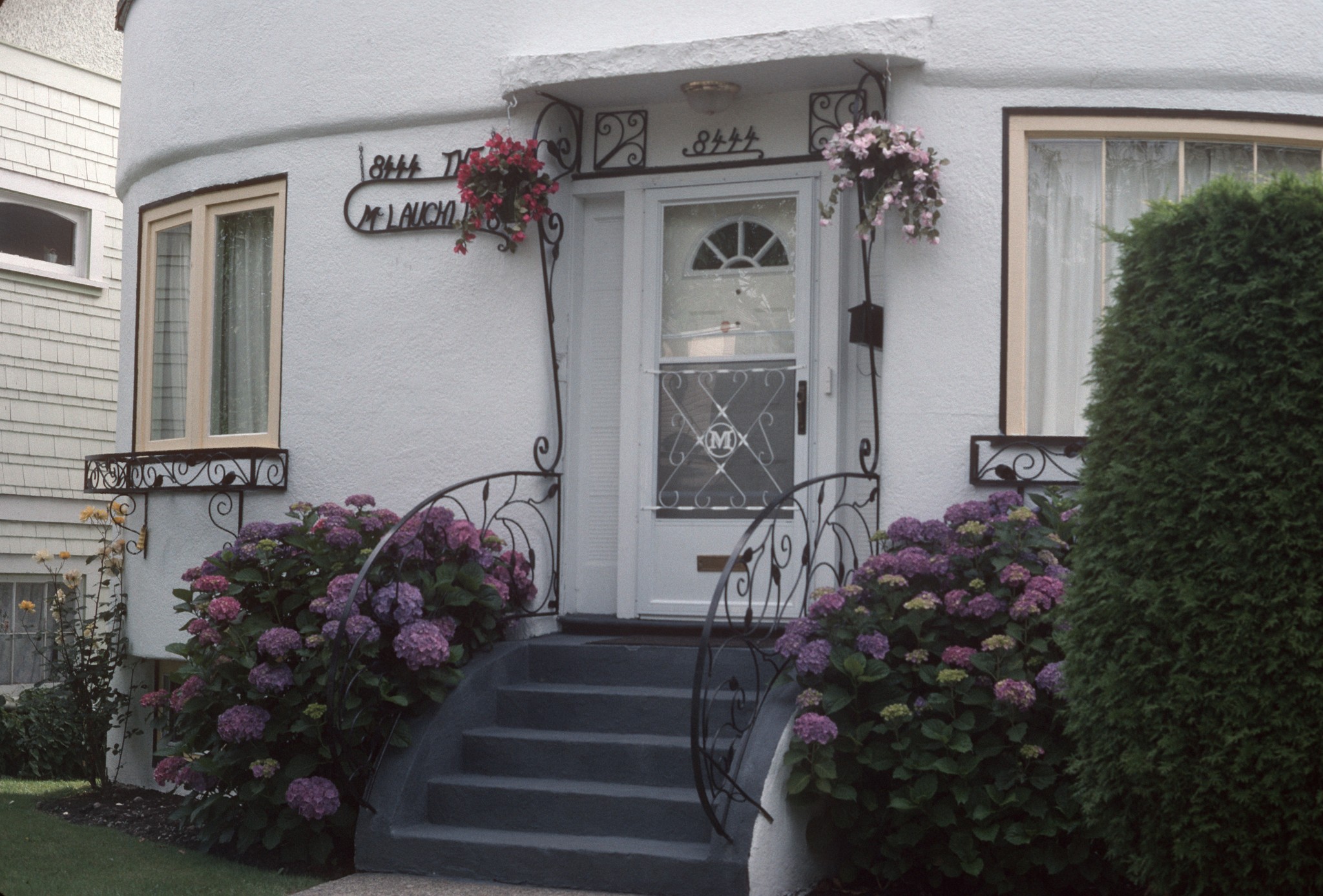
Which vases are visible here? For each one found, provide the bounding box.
[481,167,523,227]
[849,146,904,205]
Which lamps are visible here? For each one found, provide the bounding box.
[683,82,747,118]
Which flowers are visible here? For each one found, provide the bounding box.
[817,113,947,247]
[451,130,561,256]
